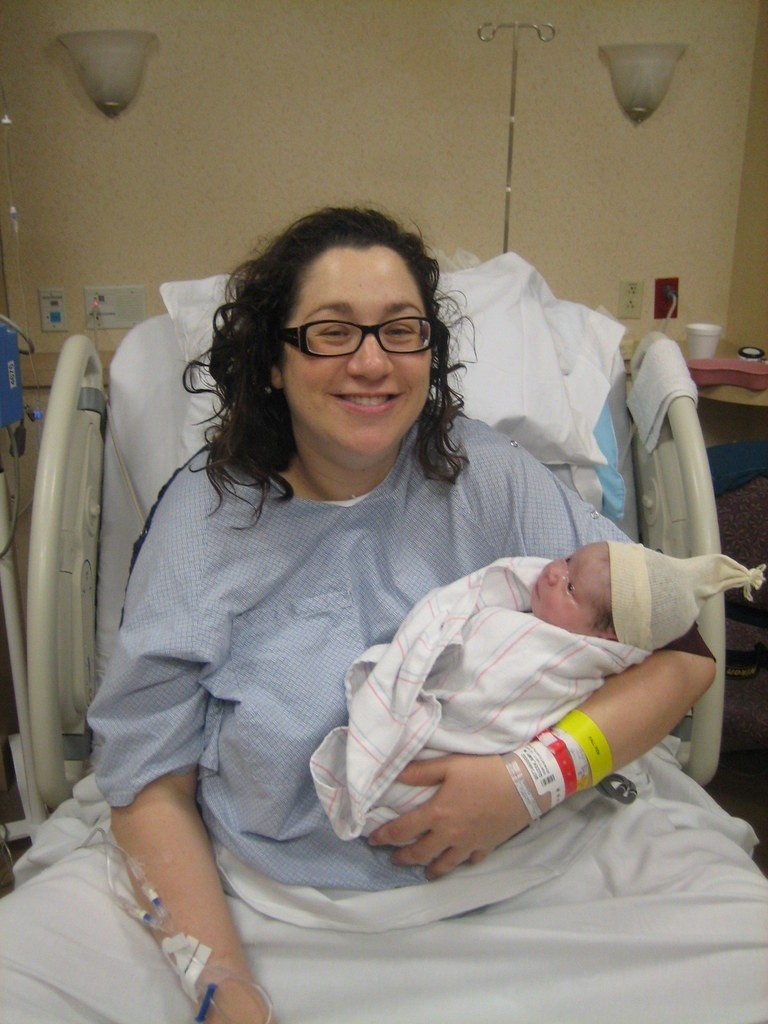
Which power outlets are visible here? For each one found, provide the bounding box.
[654,278,679,319]
[619,280,642,319]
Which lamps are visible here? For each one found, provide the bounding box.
[58,29,160,118]
[599,41,688,125]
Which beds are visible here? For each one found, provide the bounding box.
[0,205,768,1024]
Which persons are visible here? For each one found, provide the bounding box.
[84,205,715,1024]
[310,538,766,847]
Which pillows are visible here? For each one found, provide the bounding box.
[160,249,624,468]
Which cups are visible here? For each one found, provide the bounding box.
[688,323,721,360]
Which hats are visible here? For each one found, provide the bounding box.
[605,538,766,652]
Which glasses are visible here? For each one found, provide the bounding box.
[280,316,435,358]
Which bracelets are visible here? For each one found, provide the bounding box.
[505,707,615,821]
